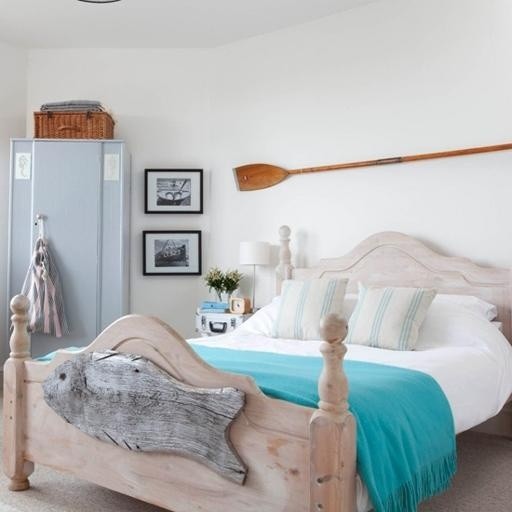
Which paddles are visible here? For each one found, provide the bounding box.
[235,144,512,190]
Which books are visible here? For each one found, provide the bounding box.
[199,301,229,313]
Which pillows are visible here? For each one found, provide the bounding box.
[264,273,354,338]
[343,283,438,352]
[421,286,499,346]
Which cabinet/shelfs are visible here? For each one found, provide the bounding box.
[6,137,133,360]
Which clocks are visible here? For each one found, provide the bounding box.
[228,298,250,315]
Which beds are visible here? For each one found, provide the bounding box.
[0,227,512,512]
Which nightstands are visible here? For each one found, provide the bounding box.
[195,311,253,337]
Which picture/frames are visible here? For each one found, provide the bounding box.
[142,230,201,276]
[144,167,203,214]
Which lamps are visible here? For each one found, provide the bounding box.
[239,242,271,314]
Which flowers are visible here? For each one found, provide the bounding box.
[204,268,243,305]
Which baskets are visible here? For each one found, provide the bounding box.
[33,112,115,139]
